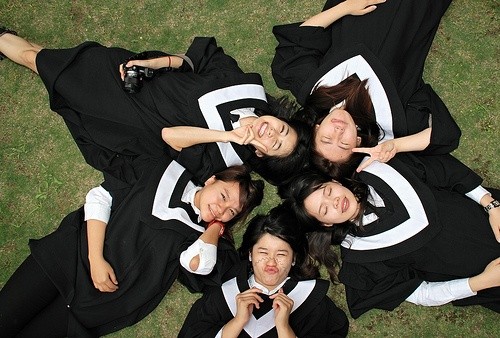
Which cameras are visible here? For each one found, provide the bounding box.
[123,64,153,95]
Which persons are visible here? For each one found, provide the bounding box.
[271,0,462,179]
[177,215,349,338]
[0,22,315,186]
[1,160,265,338]
[288,151,500,319]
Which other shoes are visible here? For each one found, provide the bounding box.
[0,25,18,61]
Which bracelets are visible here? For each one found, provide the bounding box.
[168,56,171,68]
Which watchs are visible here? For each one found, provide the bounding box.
[207,220,225,236]
[484,199,500,213]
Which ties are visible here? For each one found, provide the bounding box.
[228,113,241,123]
[363,200,386,218]
